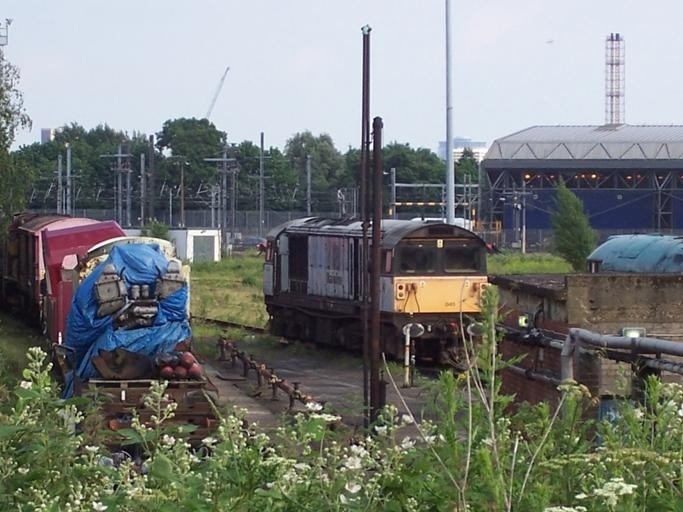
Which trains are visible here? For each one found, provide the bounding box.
[260,218,492,368]
[0,213,194,376]
